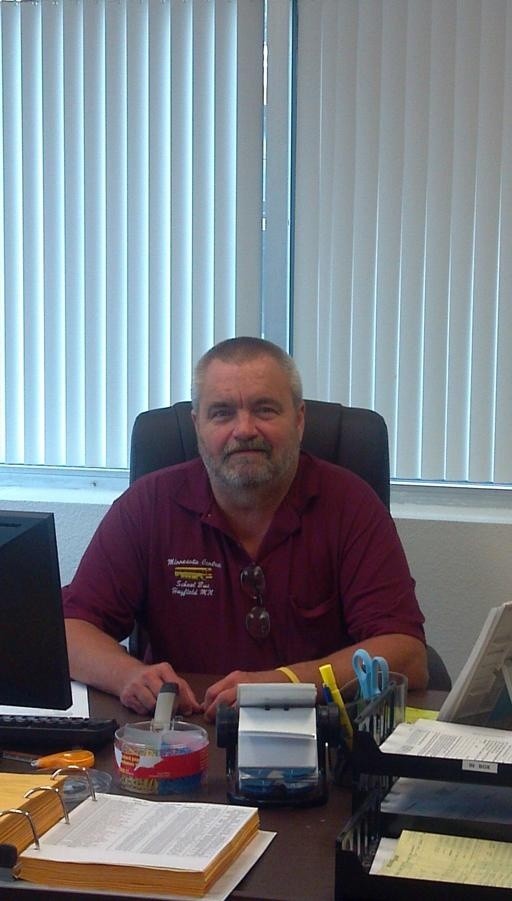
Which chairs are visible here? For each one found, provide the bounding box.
[129,400,453,691]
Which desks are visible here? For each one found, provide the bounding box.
[0,673,451,901]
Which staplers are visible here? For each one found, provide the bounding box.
[150,682,179,732]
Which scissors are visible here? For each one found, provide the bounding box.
[353,649,390,744]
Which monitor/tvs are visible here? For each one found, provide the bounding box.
[0,511,73,711]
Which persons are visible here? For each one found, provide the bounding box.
[59,336,432,726]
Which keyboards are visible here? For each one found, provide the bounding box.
[0,714,120,755]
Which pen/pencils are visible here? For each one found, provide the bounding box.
[319,664,353,755]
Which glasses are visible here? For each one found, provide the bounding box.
[239,559,273,642]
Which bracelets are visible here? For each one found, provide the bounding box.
[276,666,302,687]
